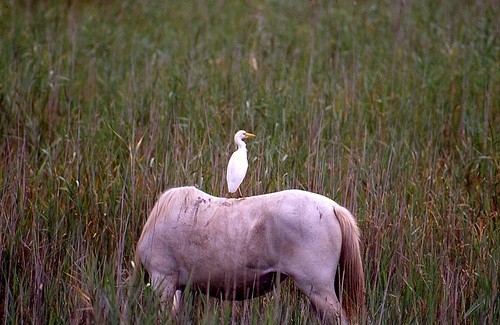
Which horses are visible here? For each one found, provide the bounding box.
[131,186,367,325]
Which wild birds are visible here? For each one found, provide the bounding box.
[226,129,256,199]
